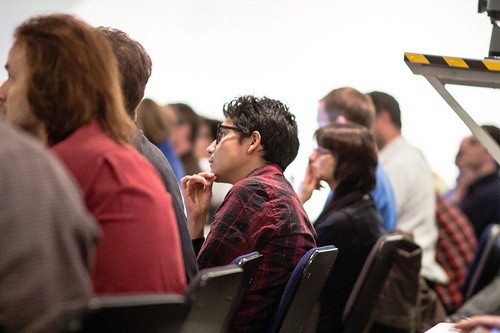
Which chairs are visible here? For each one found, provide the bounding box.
[82,224,500,333]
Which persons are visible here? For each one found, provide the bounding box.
[1,15,187,295]
[180,95,318,332]
[433,126,500,333]
[298,123,384,332]
[136,97,223,222]
[319,87,396,238]
[93,25,198,283]
[365,91,449,285]
[0,121,103,332]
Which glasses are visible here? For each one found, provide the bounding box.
[215,124,242,144]
